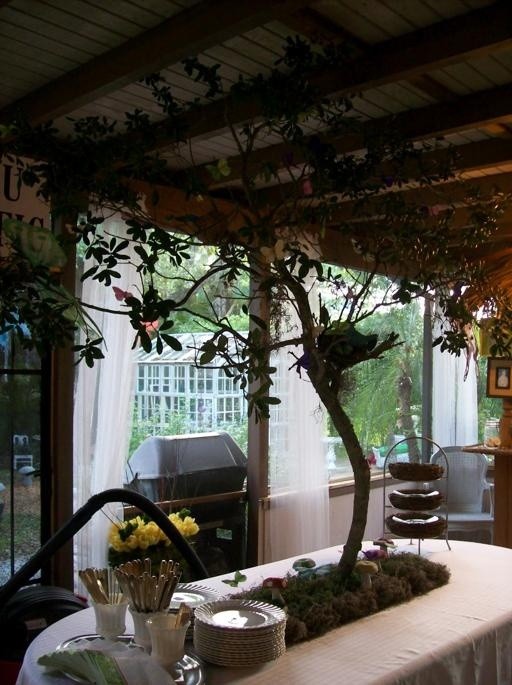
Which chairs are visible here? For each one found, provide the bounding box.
[13,434,34,469]
[422,444,497,543]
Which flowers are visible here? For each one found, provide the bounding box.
[105,509,202,570]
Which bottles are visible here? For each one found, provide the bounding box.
[483,417,501,450]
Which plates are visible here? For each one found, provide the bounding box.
[54,633,207,685]
[166,583,289,668]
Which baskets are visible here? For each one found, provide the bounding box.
[389,489,442,509]
[388,461,446,482]
[385,513,447,538]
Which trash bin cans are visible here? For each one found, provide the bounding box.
[128,430,251,506]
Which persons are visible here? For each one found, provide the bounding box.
[498,368,508,387]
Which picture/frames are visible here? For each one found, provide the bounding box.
[486,355,512,398]
